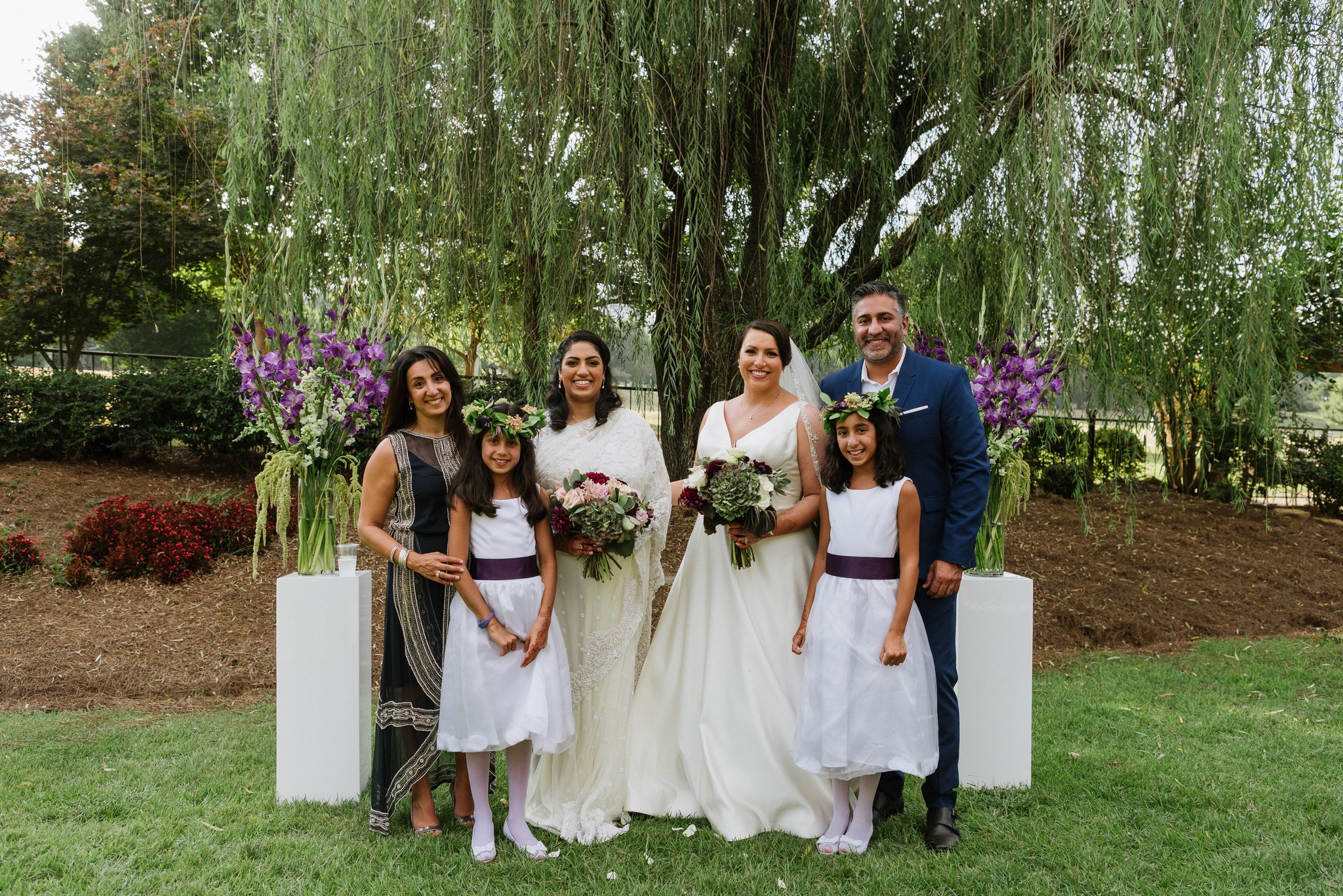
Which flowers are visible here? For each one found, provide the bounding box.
[220,299,391,579]
[911,255,1073,576]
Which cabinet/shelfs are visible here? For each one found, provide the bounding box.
[271,571,374,810]
[963,564,1035,791]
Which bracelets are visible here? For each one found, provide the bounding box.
[390,545,404,565]
[398,547,412,571]
[478,610,495,629]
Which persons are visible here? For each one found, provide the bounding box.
[663,320,849,840]
[527,327,672,848]
[353,342,498,841]
[444,397,580,865]
[789,391,945,855]
[816,277,997,851]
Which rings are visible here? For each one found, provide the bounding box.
[744,536,748,544]
[580,544,583,550]
[435,571,442,578]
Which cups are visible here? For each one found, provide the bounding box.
[336,544,358,577]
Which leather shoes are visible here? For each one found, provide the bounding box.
[927,806,963,851]
[870,792,904,827]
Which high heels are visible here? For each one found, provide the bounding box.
[411,790,443,837]
[449,775,475,829]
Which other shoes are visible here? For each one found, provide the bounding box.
[470,821,497,863]
[503,815,547,862]
[838,821,873,856]
[816,812,852,855]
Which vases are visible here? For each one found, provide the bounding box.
[294,473,335,574]
[963,485,1005,577]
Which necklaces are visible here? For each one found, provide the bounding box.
[567,408,599,430]
[741,385,781,419]
